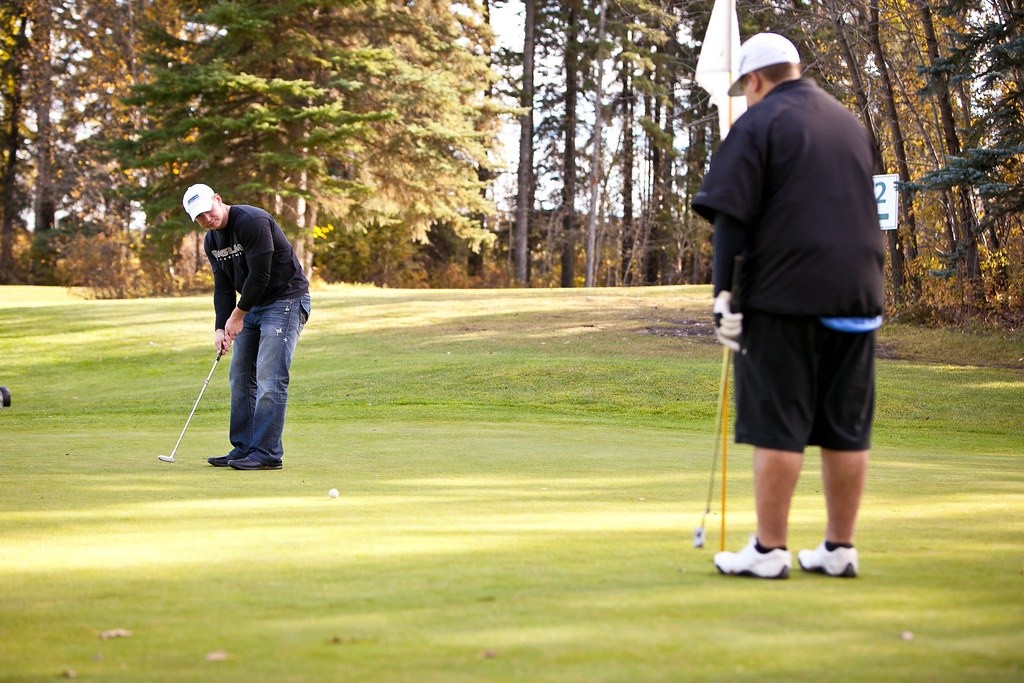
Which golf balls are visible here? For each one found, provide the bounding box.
[329,488,340,499]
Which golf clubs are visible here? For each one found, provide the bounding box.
[692,256,747,548]
[157,336,231,463]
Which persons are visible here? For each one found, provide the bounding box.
[692,31,888,579]
[183,183,310,470]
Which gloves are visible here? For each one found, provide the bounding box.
[711,290,745,353]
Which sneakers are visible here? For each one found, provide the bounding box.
[713,541,792,579]
[208,454,239,466]
[797,541,859,578]
[228,456,283,470]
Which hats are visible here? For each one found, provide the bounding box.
[183,184,215,222]
[727,33,800,97]
